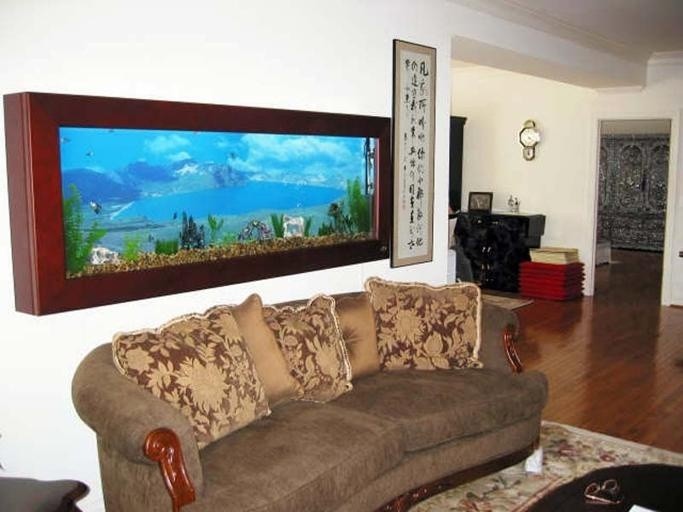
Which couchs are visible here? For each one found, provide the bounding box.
[72,290,547,511]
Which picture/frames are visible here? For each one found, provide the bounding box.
[392,39,436,268]
[468,193,492,212]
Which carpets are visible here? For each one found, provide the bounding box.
[482,293,535,310]
[409,420,683,512]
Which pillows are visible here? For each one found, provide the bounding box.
[114,304,275,451]
[365,277,484,376]
[262,294,354,407]
[329,287,381,383]
[229,291,304,410]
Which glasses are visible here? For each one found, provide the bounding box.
[585,479,626,506]
